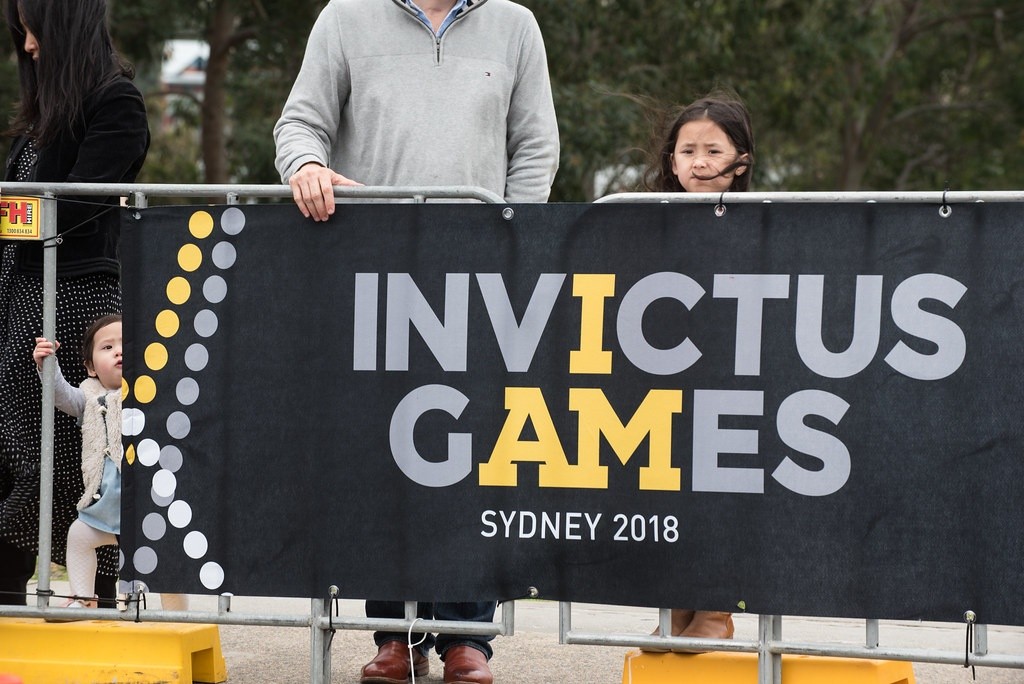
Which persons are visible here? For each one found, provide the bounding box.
[273,0,560,684]
[33,315,188,624]
[0,0,164,618]
[638,99,756,653]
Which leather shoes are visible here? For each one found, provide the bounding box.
[670,609,733,652]
[443,645,493,684]
[360,640,429,684]
[638,607,695,652]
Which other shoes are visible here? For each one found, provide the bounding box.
[44,594,99,622]
